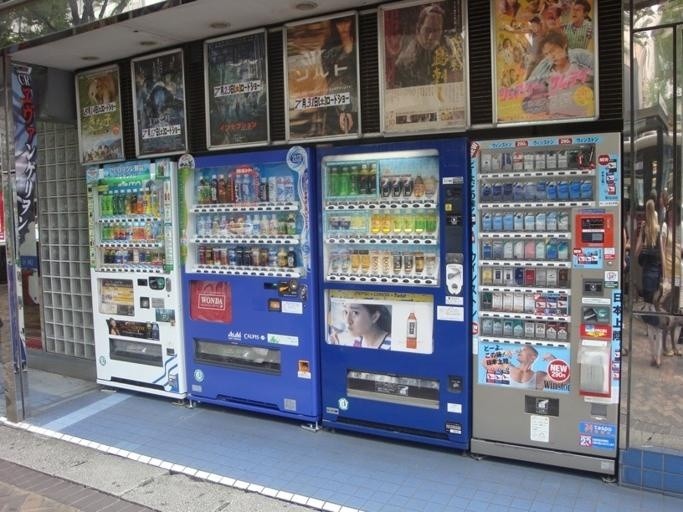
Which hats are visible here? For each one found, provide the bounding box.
[146,82,174,107]
[298,23,330,36]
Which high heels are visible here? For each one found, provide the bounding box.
[651,354,663,368]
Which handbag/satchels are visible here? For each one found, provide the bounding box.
[639,246,649,267]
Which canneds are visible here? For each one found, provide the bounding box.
[200,247,269,266]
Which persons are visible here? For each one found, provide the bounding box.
[320,16,357,137]
[481,344,566,388]
[385,9,464,88]
[623,184,681,367]
[159,61,178,97]
[325,300,390,351]
[495,2,595,116]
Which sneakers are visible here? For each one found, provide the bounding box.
[664,349,681,356]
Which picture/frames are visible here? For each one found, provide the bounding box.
[487,1,601,129]
[74,60,128,169]
[129,45,192,163]
[377,1,472,139]
[280,10,363,147]
[201,27,273,153]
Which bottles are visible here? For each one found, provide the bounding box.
[378,163,436,200]
[96,187,161,216]
[97,221,162,242]
[192,212,297,239]
[324,210,438,239]
[325,247,437,280]
[406,310,417,349]
[195,244,296,270]
[325,162,376,198]
[163,347,178,394]
[99,246,165,266]
[144,321,159,341]
[190,171,297,205]
